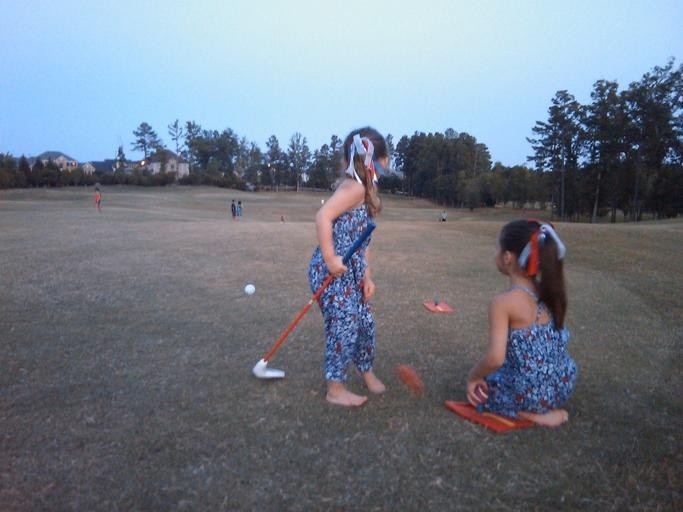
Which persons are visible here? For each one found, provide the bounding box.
[480,199,486,208]
[469,199,473,211]
[231,199,238,220]
[441,210,448,221]
[464,218,577,427]
[308,128,386,407]
[95,183,102,214]
[236,200,243,220]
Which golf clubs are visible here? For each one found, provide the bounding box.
[252,223,376,377]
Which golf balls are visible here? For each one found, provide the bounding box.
[244,284,255,295]
